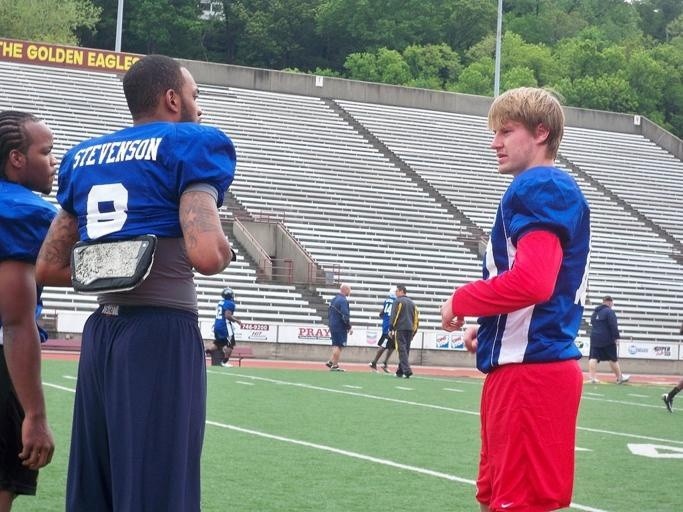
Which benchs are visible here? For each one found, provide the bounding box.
[0,61,683,341]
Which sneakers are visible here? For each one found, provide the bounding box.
[220,360,233,368]
[617,373,630,384]
[662,393,672,414]
[588,377,599,383]
[368,361,413,378]
[325,361,344,372]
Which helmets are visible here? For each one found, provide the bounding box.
[221,288,234,298]
[389,286,398,299]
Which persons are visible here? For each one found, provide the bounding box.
[589,295,629,384]
[325,283,350,372]
[205,288,244,368]
[0,110,60,512]
[661,379,683,413]
[441,84,591,512]
[369,284,419,378]
[35,54,237,512]
